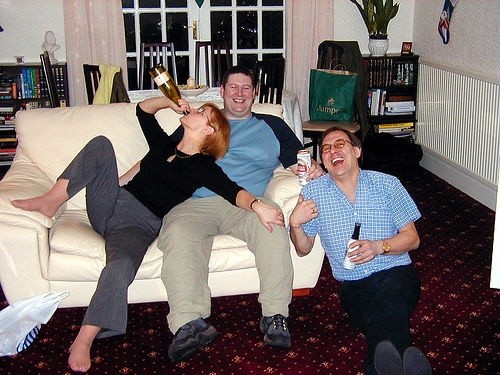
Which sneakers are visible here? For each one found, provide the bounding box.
[260,314,291,347]
[168,319,218,362]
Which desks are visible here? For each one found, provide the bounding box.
[126,86,303,147]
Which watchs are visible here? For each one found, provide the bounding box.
[379,239,391,255]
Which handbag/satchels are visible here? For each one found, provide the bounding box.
[0,290,69,357]
[358,132,424,180]
[309,58,358,122]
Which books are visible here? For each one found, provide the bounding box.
[374,122,414,136]
[0,100,51,125]
[0,125,18,166]
[368,88,387,116]
[384,100,416,116]
[369,59,414,87]
[0,67,64,99]
[60,100,66,107]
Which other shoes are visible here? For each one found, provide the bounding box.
[403,346,431,375]
[374,340,404,375]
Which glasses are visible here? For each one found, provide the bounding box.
[320,139,354,153]
[198,106,211,126]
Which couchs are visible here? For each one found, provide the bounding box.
[0,102,326,309]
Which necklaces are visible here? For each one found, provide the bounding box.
[171,145,192,158]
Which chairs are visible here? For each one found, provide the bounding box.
[83,64,129,104]
[254,58,285,104]
[40,50,60,108]
[195,40,232,88]
[302,41,371,162]
[139,43,178,89]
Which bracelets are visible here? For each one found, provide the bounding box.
[250,198,260,210]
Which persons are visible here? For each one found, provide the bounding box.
[117,64,327,360]
[288,126,433,375]
[40,30,60,54]
[11,95,285,372]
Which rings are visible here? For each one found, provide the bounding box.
[312,208,314,213]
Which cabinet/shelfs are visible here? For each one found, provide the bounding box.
[0,62,70,182]
[361,53,419,142]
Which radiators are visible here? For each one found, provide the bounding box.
[415,58,500,190]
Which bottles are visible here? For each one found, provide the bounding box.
[148,64,190,116]
[343,222,362,271]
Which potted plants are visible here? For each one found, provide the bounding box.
[350,0,400,56]
